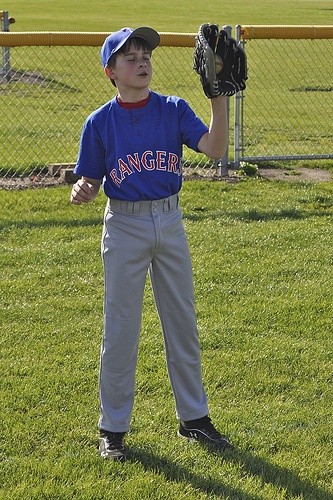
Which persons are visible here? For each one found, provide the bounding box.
[69,24,248,462]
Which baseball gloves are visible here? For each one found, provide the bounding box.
[193,23,249,98]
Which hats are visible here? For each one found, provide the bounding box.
[100,27,160,70]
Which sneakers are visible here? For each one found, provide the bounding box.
[176,416,236,455]
[96,428,126,463]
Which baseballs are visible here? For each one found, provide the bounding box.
[214,53,224,75]
